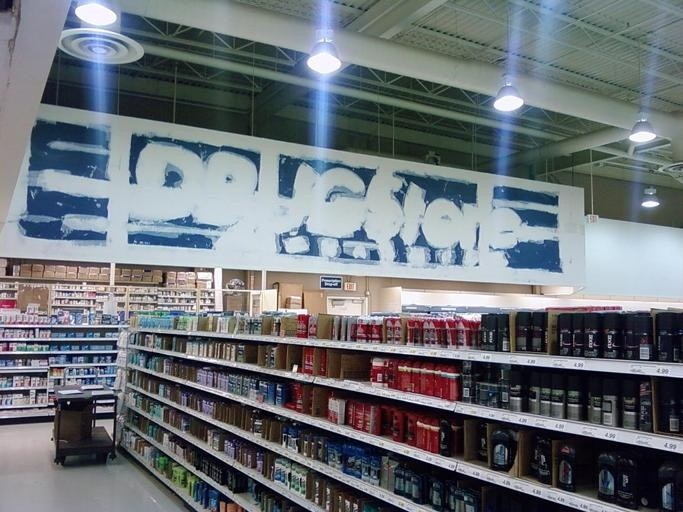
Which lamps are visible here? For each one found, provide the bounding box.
[629,37,656,143]
[494,10,524,112]
[74,1,119,27]
[306,25,343,74]
[641,174,660,208]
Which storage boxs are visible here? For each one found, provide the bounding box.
[303,290,326,315]
[272,282,304,309]
[285,296,303,309]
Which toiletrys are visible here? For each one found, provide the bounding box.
[120,304,683,512]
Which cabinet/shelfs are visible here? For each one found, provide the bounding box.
[1,255,267,420]
[112,286,681,510]
